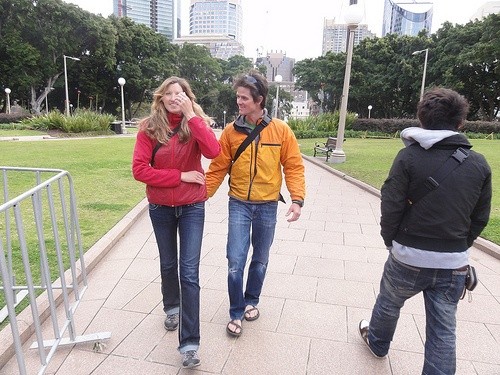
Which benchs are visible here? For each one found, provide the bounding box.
[314,137,347,162]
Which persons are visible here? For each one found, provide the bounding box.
[357,88,493,375]
[204,70,306,336]
[131,76,221,369]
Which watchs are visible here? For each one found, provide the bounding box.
[292,200,305,208]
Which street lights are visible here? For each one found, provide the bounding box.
[223,111,226,129]
[45,87,55,114]
[329,4,363,163]
[368,105,372,118]
[5,88,12,114]
[64,55,80,117]
[275,75,282,118]
[412,49,428,102]
[118,77,126,133]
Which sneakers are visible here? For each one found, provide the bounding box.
[183,350,201,368]
[164,312,179,330]
[358,318,387,359]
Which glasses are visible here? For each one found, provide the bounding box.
[245,74,258,83]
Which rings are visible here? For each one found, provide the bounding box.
[297,217,298,218]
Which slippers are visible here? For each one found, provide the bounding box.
[244,304,259,321]
[226,318,242,337]
